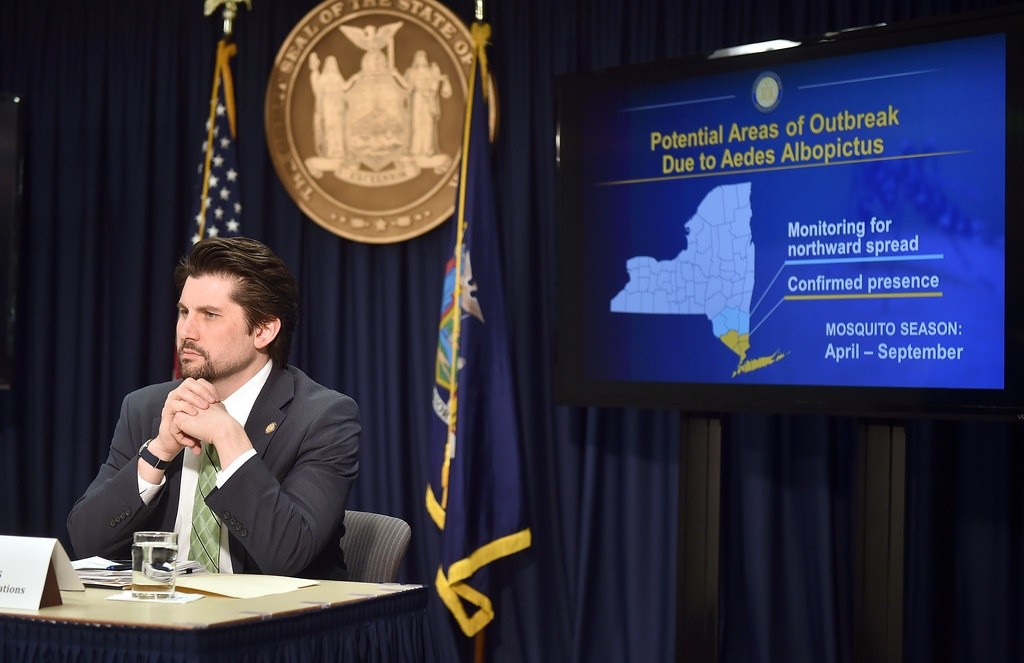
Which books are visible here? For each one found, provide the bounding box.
[74,554,205,590]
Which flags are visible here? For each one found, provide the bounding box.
[416,24,534,638]
[185,40,245,251]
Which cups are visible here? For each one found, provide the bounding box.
[131,531,178,600]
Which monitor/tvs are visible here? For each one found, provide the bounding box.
[557,13,1024,423]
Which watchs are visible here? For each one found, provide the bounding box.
[138,439,171,470]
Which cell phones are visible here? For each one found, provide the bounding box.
[106,560,132,571]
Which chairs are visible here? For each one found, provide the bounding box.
[312,511,411,583]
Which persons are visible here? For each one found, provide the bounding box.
[66,236,362,582]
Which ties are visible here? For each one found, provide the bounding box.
[187,443,222,574]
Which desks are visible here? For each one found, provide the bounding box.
[0,557,443,663]
